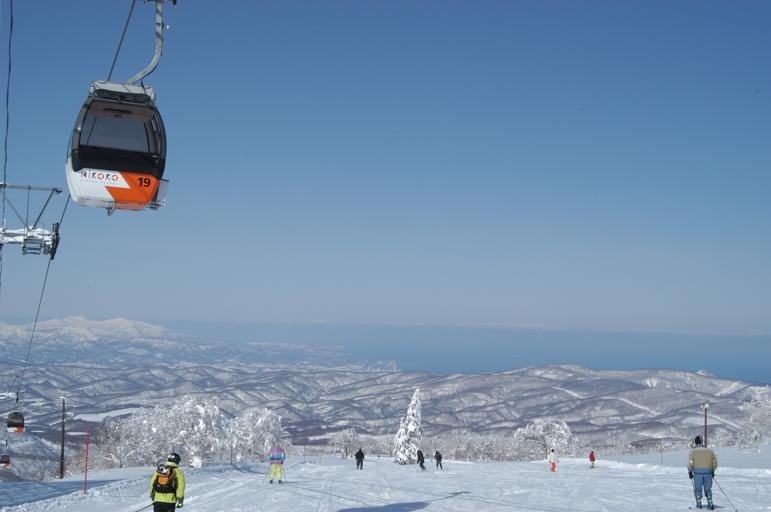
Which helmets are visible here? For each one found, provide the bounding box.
[167,453,181,464]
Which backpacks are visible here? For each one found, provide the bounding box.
[153,464,177,493]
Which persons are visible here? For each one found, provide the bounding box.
[150,452,185,512]
[417,449,427,471]
[687,435,718,510]
[356,448,365,470]
[268,441,286,484]
[588,451,596,468]
[434,450,443,470]
[549,449,557,472]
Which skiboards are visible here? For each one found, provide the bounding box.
[689,506,738,511]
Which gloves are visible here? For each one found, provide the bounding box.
[176,500,184,508]
[712,472,714,477]
[688,471,693,479]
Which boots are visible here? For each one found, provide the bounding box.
[695,497,702,507]
[708,497,714,508]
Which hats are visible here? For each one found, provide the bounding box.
[695,435,703,444]
